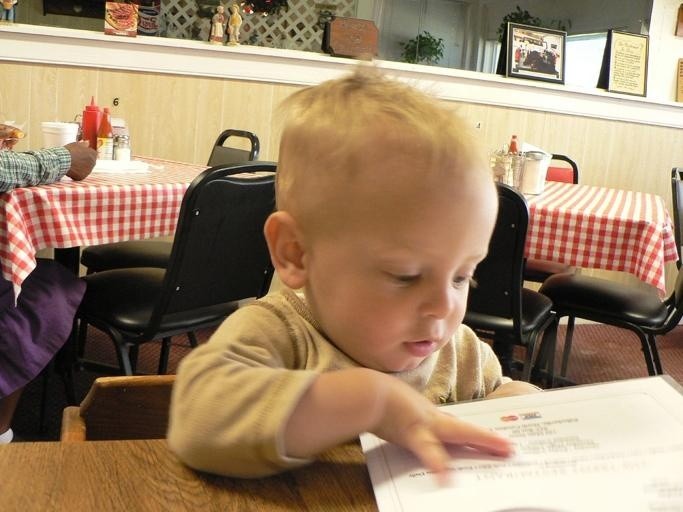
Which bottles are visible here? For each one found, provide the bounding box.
[489,151,553,195]
[508,132,521,153]
[74,95,131,162]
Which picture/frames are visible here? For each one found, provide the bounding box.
[608,29,648,97]
[506,22,565,84]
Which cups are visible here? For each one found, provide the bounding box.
[41,121,77,154]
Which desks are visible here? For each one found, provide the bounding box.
[0,440,378,512]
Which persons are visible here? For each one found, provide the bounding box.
[0,124,99,442]
[209,4,242,47]
[165,65,544,480]
